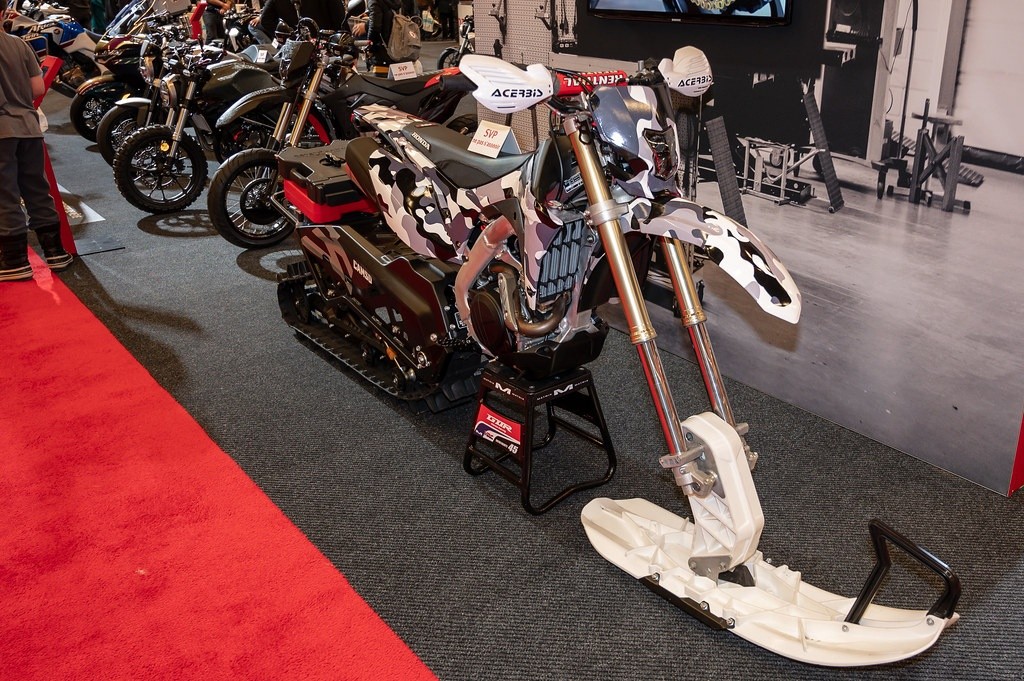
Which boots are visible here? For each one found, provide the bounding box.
[0,233,33,281]
[33,222,73,271]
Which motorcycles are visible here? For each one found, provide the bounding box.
[262,48,969,670]
[0,0,490,248]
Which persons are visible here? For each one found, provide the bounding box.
[436,0,459,41]
[0,1,72,281]
[367,0,409,78]
[417,0,437,41]
[198,0,237,44]
[248,0,368,45]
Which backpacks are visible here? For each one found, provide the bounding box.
[379,6,423,61]
